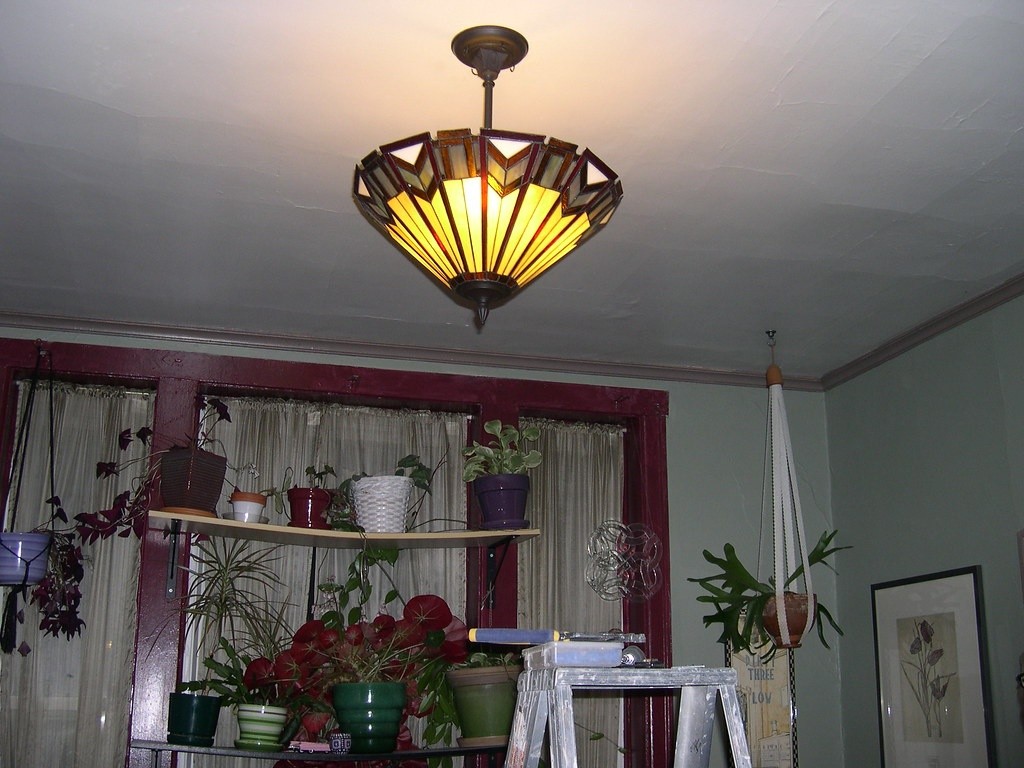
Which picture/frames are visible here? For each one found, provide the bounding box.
[726,608,799,768]
[870,565,998,768]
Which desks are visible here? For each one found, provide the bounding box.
[504,666,751,767]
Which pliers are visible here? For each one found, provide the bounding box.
[469,628,646,644]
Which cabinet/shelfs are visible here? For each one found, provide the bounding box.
[131,510,541,768]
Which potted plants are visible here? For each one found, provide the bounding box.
[461,418,543,521]
[73,393,232,542]
[177,638,333,745]
[447,652,520,737]
[287,464,337,529]
[230,484,282,523]
[167,637,238,738]
[337,454,432,533]
[0,494,87,656]
[688,528,853,664]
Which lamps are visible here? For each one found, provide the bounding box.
[353,24,625,334]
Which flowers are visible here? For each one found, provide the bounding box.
[245,595,465,749]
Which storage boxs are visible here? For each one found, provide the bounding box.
[521,641,624,670]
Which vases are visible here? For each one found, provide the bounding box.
[333,680,404,752]
